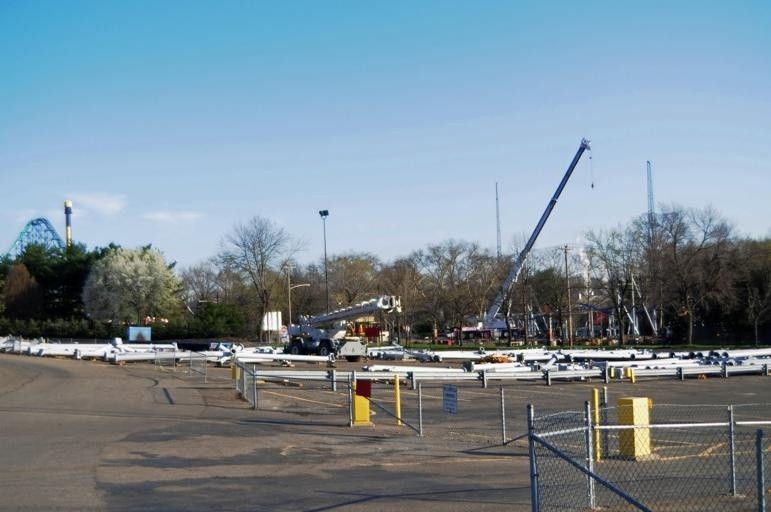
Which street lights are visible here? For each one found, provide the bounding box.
[283,265,312,324]
[319,209,330,315]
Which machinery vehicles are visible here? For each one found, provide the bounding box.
[451,137,596,338]
[284,294,404,365]
